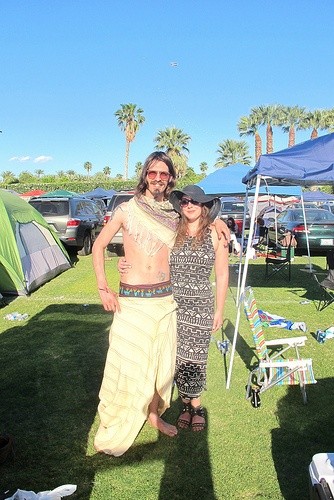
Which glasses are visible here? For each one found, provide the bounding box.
[147,169,172,181]
[179,198,199,207]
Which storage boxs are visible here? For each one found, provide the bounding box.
[276,242,294,260]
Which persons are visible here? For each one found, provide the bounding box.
[118,185,229,433]
[226,218,240,237]
[92,152,231,457]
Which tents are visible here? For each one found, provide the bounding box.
[0,189,71,297]
[194,132,334,390]
[19,187,117,200]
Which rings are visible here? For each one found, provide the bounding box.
[221,325,222,328]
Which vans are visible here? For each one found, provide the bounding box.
[219,197,249,230]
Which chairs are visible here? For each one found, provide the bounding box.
[239,285,319,408]
[313,250,334,311]
[265,234,293,282]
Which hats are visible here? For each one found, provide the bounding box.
[168,184,221,223]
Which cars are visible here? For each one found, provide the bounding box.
[103,191,135,255]
[268,208,334,252]
[287,200,334,214]
[28,198,106,256]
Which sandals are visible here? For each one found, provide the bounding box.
[190,407,207,432]
[177,402,192,429]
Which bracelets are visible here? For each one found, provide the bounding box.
[99,286,108,293]
